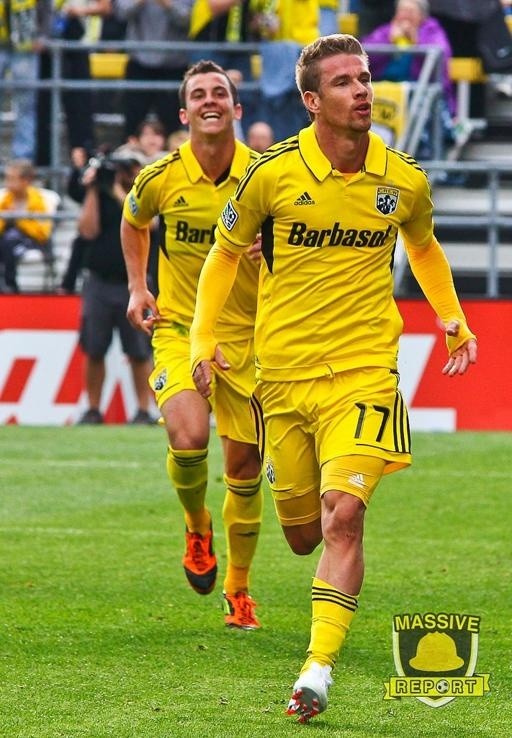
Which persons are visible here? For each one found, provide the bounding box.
[1,0,511,296]
[120,61,277,628]
[78,155,163,424]
[188,34,477,719]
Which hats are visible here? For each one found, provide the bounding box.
[407,631,466,675]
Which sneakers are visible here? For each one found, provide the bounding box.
[132,409,158,424]
[79,407,105,424]
[180,507,221,596]
[284,659,335,726]
[219,589,263,633]
